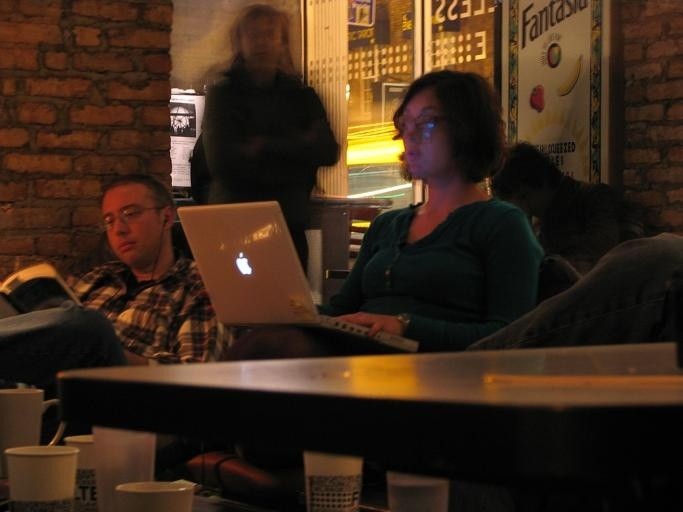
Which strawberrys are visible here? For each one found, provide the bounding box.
[530,85,546,111]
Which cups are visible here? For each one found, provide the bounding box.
[303,452,363,512]
[64,435,99,507]
[113,480,197,512]
[0,388,65,447]
[4,445,81,511]
[386,471,451,512]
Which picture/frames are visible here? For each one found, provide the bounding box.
[493,1,624,196]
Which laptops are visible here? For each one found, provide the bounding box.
[175,198,419,353]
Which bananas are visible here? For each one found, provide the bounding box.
[556,53,584,96]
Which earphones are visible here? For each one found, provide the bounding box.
[164,219,169,224]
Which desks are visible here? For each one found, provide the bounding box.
[58,340,681,511]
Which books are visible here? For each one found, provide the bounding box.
[0,262,82,313]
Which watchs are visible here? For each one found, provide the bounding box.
[397,312,411,334]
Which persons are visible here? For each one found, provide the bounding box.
[203,4,341,277]
[315,71,542,356]
[493,142,650,301]
[0,172,225,367]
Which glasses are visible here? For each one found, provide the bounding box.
[396,112,444,138]
[99,205,159,230]
